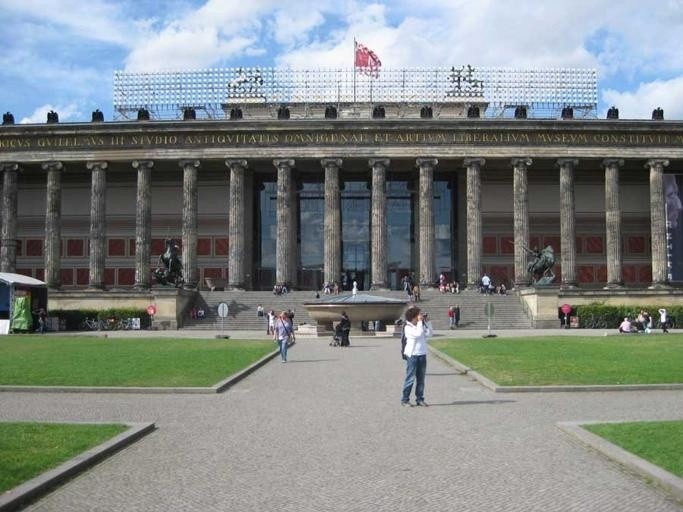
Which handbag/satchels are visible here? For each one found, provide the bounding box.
[287,335,292,344]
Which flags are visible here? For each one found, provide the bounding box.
[354,41,381,80]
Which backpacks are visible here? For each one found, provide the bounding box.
[401,322,413,360]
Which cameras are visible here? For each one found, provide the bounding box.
[420,313,427,317]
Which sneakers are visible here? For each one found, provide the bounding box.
[281,359,287,363]
[401,401,414,407]
[416,402,428,407]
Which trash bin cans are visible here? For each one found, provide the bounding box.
[570,316,580,328]
[244,273,255,291]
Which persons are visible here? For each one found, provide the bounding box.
[272,271,508,302]
[663,174,683,231]
[618,307,672,334]
[529,247,541,272]
[256,303,264,317]
[400,307,434,408]
[453,303,460,327]
[197,306,204,319]
[338,311,350,347]
[272,310,294,363]
[33,308,45,333]
[189,306,196,320]
[267,310,274,335]
[447,306,454,327]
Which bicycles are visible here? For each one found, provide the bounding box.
[80,317,131,330]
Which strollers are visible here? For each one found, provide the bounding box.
[329,326,343,346]
